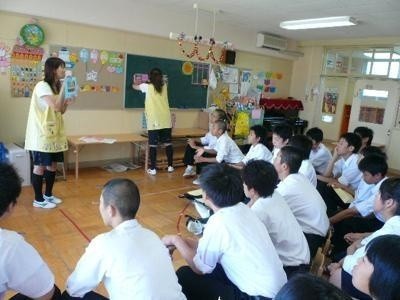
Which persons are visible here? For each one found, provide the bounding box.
[131,67,175,175]
[0,163,61,300]
[61,178,187,300]
[161,109,399,300]
[24,57,75,209]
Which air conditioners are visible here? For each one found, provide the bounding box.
[256,33,288,52]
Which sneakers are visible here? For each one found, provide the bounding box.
[32,199,57,208]
[43,195,63,204]
[147,165,200,185]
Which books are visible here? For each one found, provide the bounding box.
[100,161,142,173]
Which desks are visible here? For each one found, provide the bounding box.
[141,127,208,168]
[66,132,149,179]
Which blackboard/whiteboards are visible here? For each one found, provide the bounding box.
[123,52,212,110]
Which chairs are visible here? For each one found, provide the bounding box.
[325,229,332,254]
[311,247,325,278]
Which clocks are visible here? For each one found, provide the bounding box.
[20,23,45,47]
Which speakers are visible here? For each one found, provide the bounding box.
[225,50,235,65]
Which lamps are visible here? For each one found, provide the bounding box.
[278,16,358,30]
